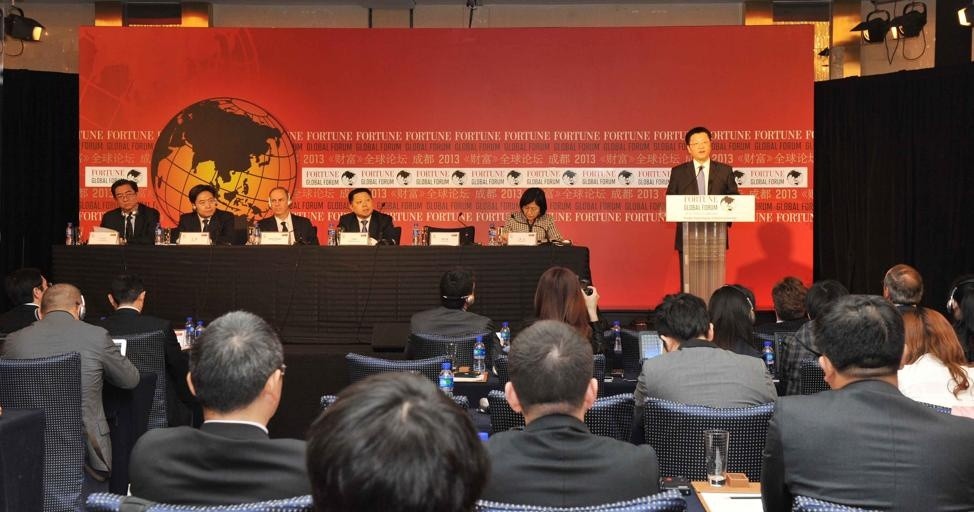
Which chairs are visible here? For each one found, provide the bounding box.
[343,352,452,387]
[608,327,660,380]
[403,328,493,374]
[109,330,169,431]
[425,226,475,244]
[643,395,775,483]
[495,354,607,394]
[483,389,633,445]
[2,352,90,512]
[472,486,689,512]
[85,490,313,512]
[791,496,880,512]
[801,360,832,395]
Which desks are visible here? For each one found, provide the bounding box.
[52,246,589,344]
[101,370,159,497]
[1,405,51,511]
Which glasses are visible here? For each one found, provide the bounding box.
[689,141,709,147]
[116,193,135,198]
[195,197,217,206]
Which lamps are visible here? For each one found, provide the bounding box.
[889,1,928,37]
[850,1,889,43]
[1,2,43,58]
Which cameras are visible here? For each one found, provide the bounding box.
[580,279,593,296]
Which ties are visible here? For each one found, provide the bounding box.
[361,221,368,233]
[527,220,535,232]
[697,167,705,195]
[202,220,209,232]
[281,222,288,232]
[126,216,133,238]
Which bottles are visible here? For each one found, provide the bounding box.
[474,337,485,374]
[154,223,164,246]
[500,323,510,348]
[437,363,455,399]
[196,321,203,338]
[185,317,195,346]
[328,224,336,246]
[762,340,775,378]
[252,223,262,245]
[412,224,420,245]
[488,224,497,246]
[612,321,622,350]
[66,223,75,245]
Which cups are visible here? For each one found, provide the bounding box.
[335,226,345,246]
[420,226,429,247]
[703,429,730,489]
[496,226,505,246]
[443,341,457,371]
[246,223,257,245]
[162,226,171,244]
[74,225,85,246]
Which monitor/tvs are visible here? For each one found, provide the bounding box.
[112,338,127,357]
[638,330,664,364]
[174,329,186,350]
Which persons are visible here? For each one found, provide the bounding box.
[780,281,849,396]
[481,319,660,509]
[306,370,491,512]
[100,179,160,245]
[947,278,973,365]
[883,264,924,305]
[93,274,196,418]
[404,266,493,357]
[129,310,312,507]
[502,189,564,245]
[176,184,234,244]
[752,277,808,334]
[0,269,52,341]
[1,283,140,482]
[254,187,316,245]
[633,293,778,430]
[708,284,767,366]
[334,188,394,244]
[760,294,974,512]
[534,267,606,355]
[897,305,973,420]
[665,127,740,293]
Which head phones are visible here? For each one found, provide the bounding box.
[34,293,86,321]
[947,279,974,321]
[442,295,475,306]
[722,285,756,325]
[189,185,217,211]
[268,187,293,207]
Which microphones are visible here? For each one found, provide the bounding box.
[510,213,550,241]
[374,203,385,243]
[456,213,467,227]
[681,166,704,194]
